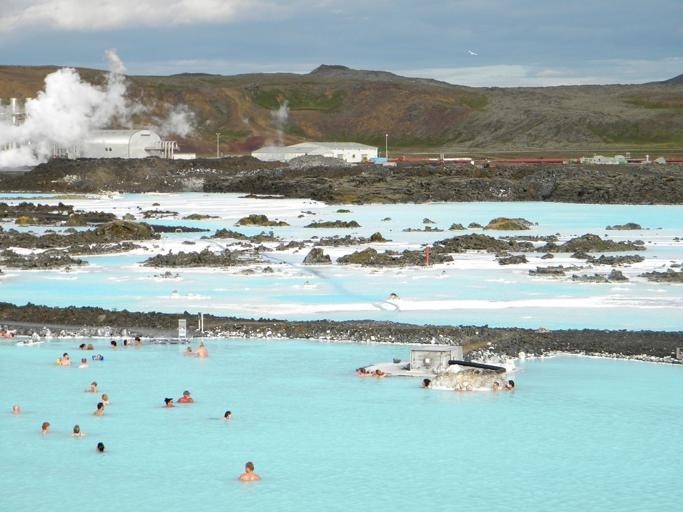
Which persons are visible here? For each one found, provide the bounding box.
[353,366,386,379]
[223,410,233,420]
[181,342,210,358]
[8,402,21,415]
[418,377,432,391]
[38,419,85,439]
[94,441,105,452]
[490,381,518,392]
[237,461,262,482]
[161,390,194,408]
[82,382,113,418]
[0,328,14,339]
[48,335,144,370]
